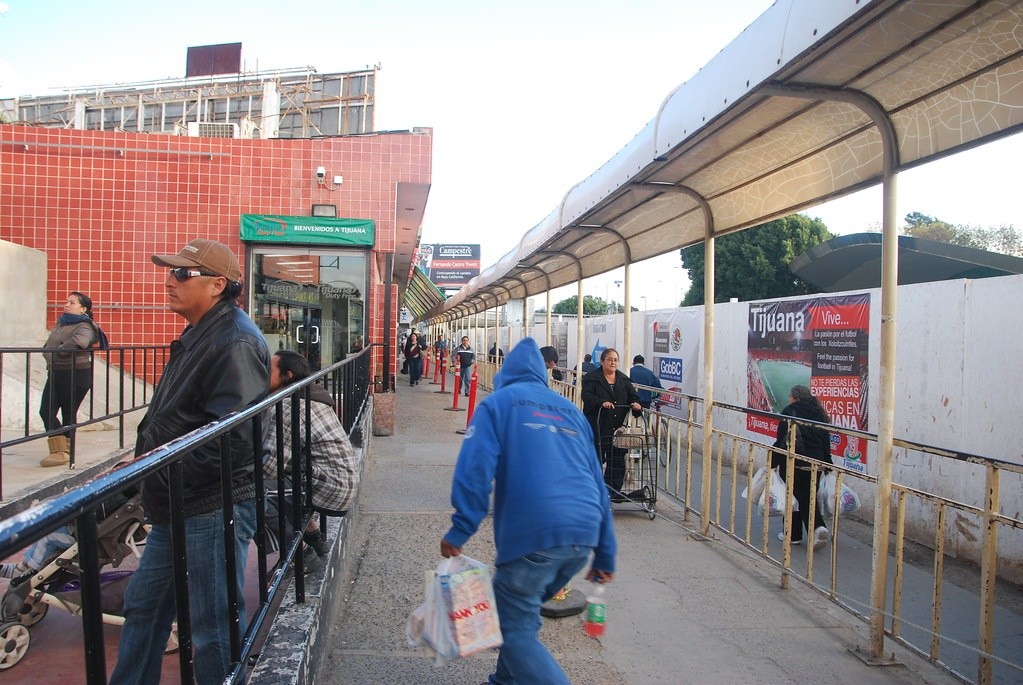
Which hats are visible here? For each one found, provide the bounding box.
[151,238,242,284]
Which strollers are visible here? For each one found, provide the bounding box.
[1,460,181,674]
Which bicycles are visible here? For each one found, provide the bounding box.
[641,394,673,468]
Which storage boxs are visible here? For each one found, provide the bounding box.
[429,567,504,656]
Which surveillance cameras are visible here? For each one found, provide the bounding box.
[317,166,326,178]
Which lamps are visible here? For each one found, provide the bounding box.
[312,203,336,217]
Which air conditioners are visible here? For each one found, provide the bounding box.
[187,121,239,138]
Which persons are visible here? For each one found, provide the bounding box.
[771,385,833,551]
[452,335,475,396]
[354,336,362,351]
[0,461,139,578]
[573,354,597,388]
[540,346,559,369]
[399,328,456,387]
[263,350,359,583]
[630,356,661,458]
[41,290,109,467]
[441,336,617,685]
[489,342,504,364]
[109,239,272,685]
[582,349,642,502]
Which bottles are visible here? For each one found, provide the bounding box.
[585,576,606,636]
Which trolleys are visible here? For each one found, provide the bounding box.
[596,405,660,521]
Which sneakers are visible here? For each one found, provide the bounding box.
[267,544,322,582]
[302,529,324,556]
[812,525,829,552]
[778,532,803,544]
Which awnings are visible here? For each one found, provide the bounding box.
[404,263,447,318]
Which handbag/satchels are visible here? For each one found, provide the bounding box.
[742,465,799,517]
[613,407,652,449]
[406,554,505,668]
[816,470,861,521]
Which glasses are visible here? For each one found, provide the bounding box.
[170,267,220,283]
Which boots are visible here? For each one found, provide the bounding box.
[41,436,70,467]
